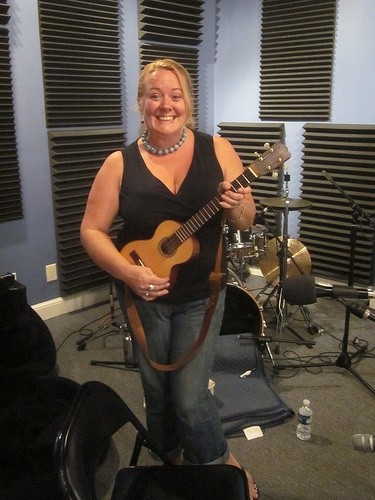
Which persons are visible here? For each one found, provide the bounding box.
[80,59,261,500]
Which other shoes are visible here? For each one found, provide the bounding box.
[251,483,257,500]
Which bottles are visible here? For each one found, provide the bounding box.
[295,399,313,440]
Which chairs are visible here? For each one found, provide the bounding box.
[54,381,250,500]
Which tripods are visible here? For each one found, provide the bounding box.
[251,169,375,396]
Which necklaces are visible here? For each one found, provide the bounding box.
[141,127,186,155]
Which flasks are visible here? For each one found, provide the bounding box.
[123,324,132,362]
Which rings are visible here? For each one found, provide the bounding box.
[148,284,155,291]
[145,291,150,296]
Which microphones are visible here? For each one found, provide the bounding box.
[351,433,375,454]
[281,274,375,307]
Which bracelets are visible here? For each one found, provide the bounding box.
[227,201,246,224]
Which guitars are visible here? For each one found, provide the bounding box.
[120,139,291,299]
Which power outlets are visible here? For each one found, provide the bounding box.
[45,263,57,283]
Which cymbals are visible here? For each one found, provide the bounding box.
[259,198,313,211]
[259,236,311,288]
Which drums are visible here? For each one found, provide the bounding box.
[220,267,266,337]
[226,242,256,261]
